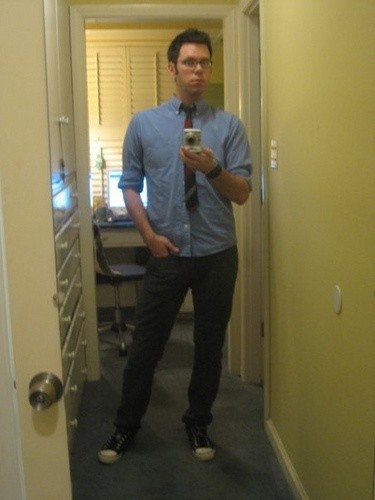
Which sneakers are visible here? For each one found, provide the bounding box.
[185,425,216,460]
[97,427,133,466]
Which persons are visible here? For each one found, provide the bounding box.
[97,28,253,465]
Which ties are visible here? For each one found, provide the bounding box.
[179,104,199,206]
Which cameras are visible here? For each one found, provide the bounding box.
[183,128,202,153]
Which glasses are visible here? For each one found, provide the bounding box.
[172,57,214,68]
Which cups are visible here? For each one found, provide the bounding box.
[93,195,104,217]
[95,208,107,223]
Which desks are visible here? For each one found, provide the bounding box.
[93,215,147,248]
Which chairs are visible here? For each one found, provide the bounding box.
[91,218,147,357]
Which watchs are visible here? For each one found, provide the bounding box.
[205,163,222,180]
